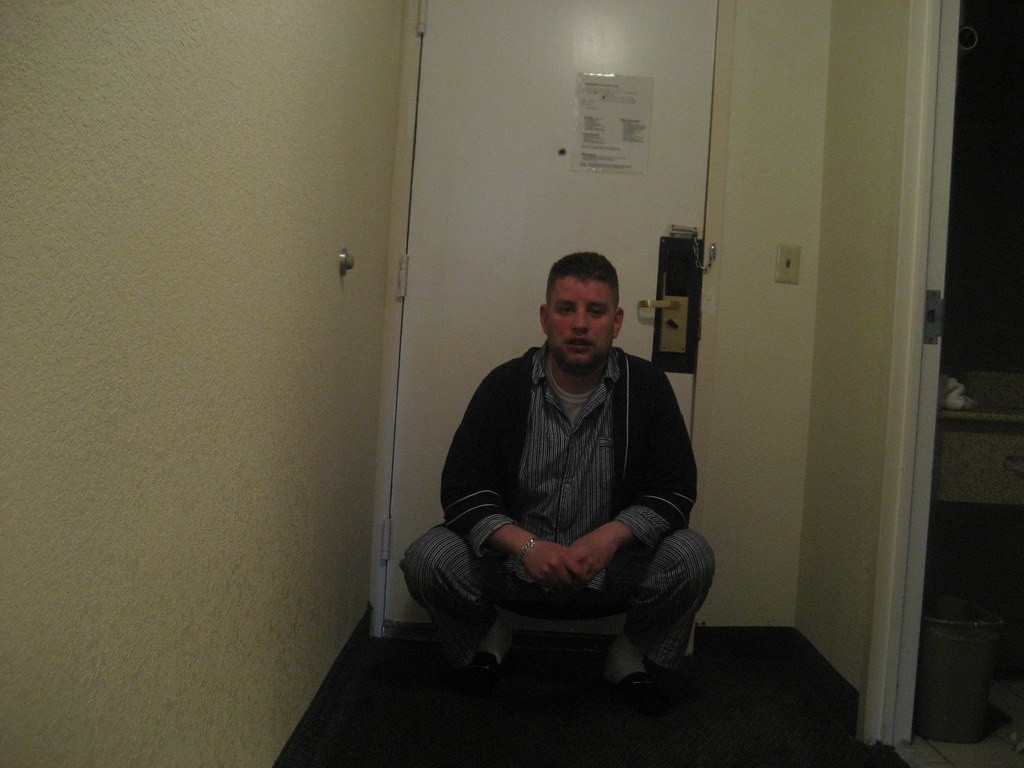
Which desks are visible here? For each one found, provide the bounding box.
[930,371,1024,505]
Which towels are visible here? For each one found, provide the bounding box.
[935,372,985,412]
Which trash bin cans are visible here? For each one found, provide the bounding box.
[913,601,1003,744]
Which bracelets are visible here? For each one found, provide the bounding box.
[515,535,542,565]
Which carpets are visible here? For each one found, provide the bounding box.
[272,601,910,768]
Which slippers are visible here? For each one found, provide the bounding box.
[449,648,511,695]
[618,670,669,716]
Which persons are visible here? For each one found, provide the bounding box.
[398,252,716,703]
